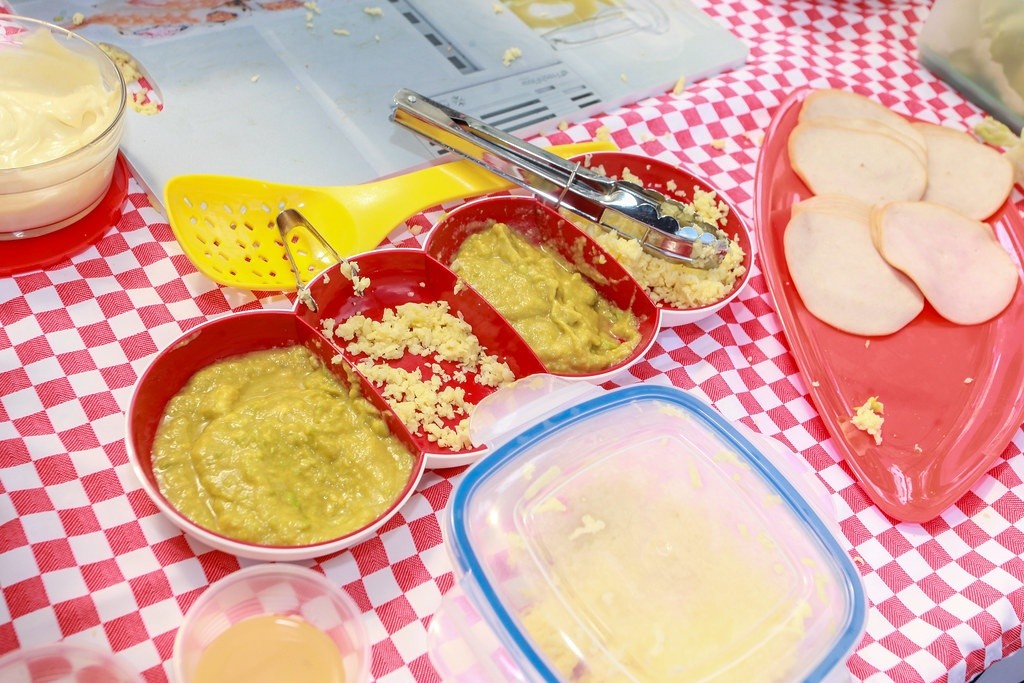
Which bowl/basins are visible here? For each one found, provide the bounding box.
[0,642,147,683]
[179,564,373,683]
[0,15,127,239]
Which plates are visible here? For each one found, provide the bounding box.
[919,0,1024,136]
[757,86,1024,520]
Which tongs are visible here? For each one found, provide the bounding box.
[273,210,363,310]
[390,91,721,267]
